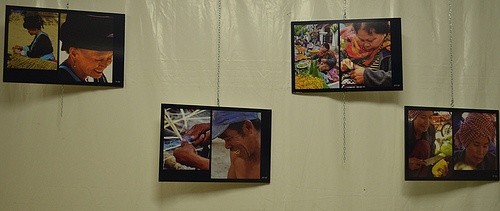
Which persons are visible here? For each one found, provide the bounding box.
[11,13,57,62]
[408,110,437,159]
[173,120,211,170]
[453,111,497,172]
[60,11,114,83]
[317,42,340,85]
[340,20,394,85]
[212,110,262,179]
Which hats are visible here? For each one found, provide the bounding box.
[212,110,258,139]
[60,14,114,52]
[453,113,497,149]
[22,15,43,31]
